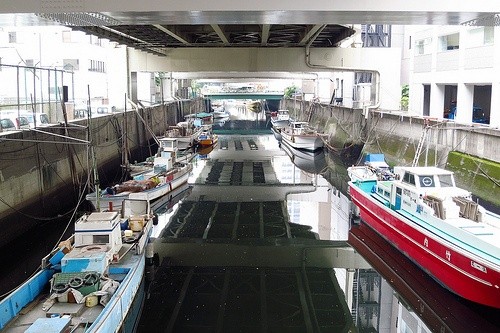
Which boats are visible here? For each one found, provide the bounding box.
[157,118,212,151]
[85,137,194,216]
[129,137,198,178]
[0,193,153,333]
[184,112,213,130]
[346,153,500,308]
[270,109,292,139]
[197,130,219,144]
[280,121,325,151]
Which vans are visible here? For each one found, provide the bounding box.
[13,116,31,129]
[0,117,16,133]
[75,109,88,119]
[28,113,50,126]
[94,106,108,115]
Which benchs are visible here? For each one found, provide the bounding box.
[22,318,72,333]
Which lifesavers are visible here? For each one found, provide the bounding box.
[125,236,136,243]
[85,273,99,285]
[69,277,83,288]
[52,283,69,294]
